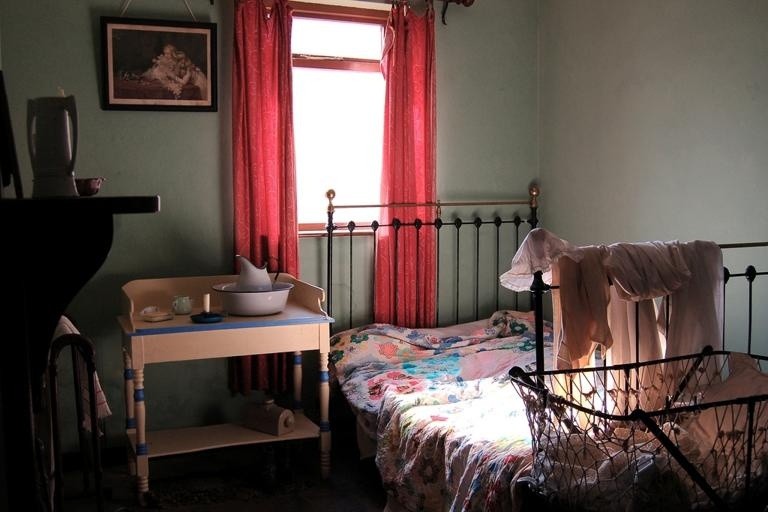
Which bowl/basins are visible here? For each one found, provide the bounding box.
[212,279,295,317]
[74,176,106,197]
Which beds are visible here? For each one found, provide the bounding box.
[319,192,768,512]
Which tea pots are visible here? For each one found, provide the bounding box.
[235,254,281,292]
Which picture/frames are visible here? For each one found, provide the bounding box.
[100,14,218,112]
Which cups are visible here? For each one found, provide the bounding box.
[171,294,195,315]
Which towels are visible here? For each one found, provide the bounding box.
[48,313,112,437]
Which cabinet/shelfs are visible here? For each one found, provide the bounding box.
[116,272,335,505]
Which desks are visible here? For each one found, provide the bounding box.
[3,192,161,508]
